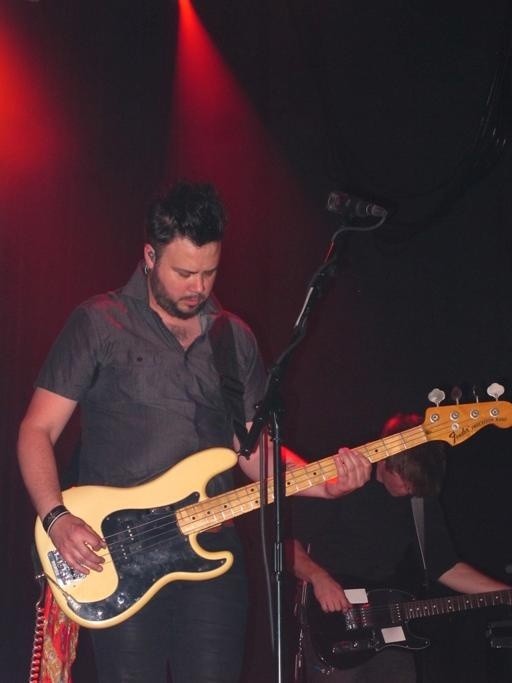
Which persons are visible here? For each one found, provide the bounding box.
[17,184,374,683]
[280,411,510,682]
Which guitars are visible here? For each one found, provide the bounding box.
[308,587,511,670]
[36,381,511,630]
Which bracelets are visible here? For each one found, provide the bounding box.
[42,504,69,536]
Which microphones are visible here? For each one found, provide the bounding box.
[325,191,388,217]
